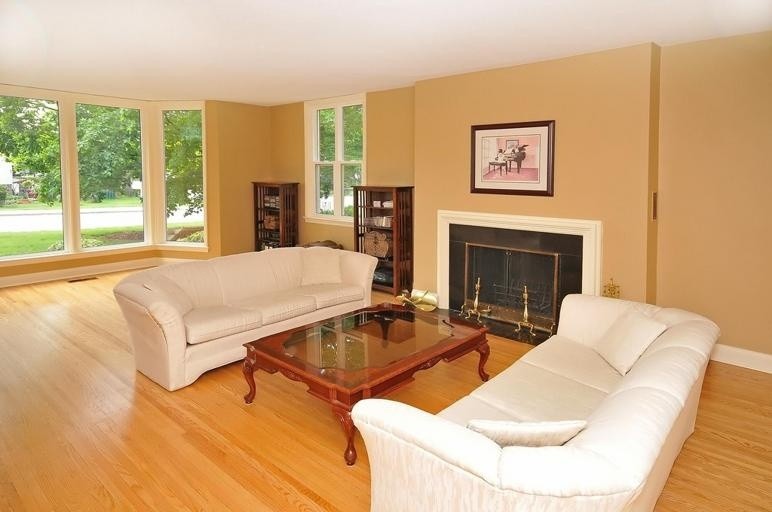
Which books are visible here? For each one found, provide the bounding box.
[360,200,394,229]
[256,194,286,250]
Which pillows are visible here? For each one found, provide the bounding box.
[591,307,667,376]
[299,245,343,287]
[143,272,194,317]
[466,417,589,447]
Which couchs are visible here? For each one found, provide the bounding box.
[113,245,378,391]
[352,292,720,512]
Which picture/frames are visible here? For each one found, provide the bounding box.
[470,119,556,197]
[506,139,519,151]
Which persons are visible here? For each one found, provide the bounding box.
[495,148,507,166]
[511,144,520,154]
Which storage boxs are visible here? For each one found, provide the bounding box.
[363,230,394,258]
[263,215,280,230]
[263,194,281,210]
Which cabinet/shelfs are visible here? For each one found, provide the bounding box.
[352,185,413,298]
[351,303,416,345]
[252,180,300,252]
